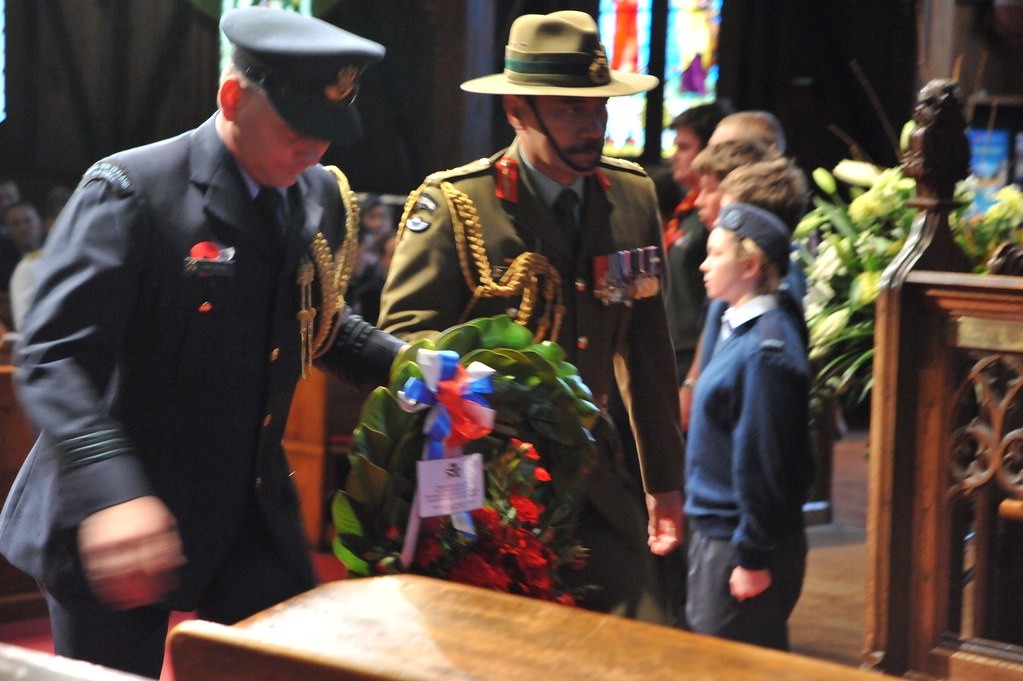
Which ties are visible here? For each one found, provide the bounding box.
[254,188,286,276]
[555,186,580,255]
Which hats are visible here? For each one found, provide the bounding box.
[220,7,385,146]
[711,203,789,278]
[460,10,659,97]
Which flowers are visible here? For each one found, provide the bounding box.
[422,435,588,606]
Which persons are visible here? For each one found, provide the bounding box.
[658,98,814,653]
[0,168,407,328]
[377,12,685,633]
[0,6,416,680]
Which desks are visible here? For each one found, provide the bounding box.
[197,572,926,681]
